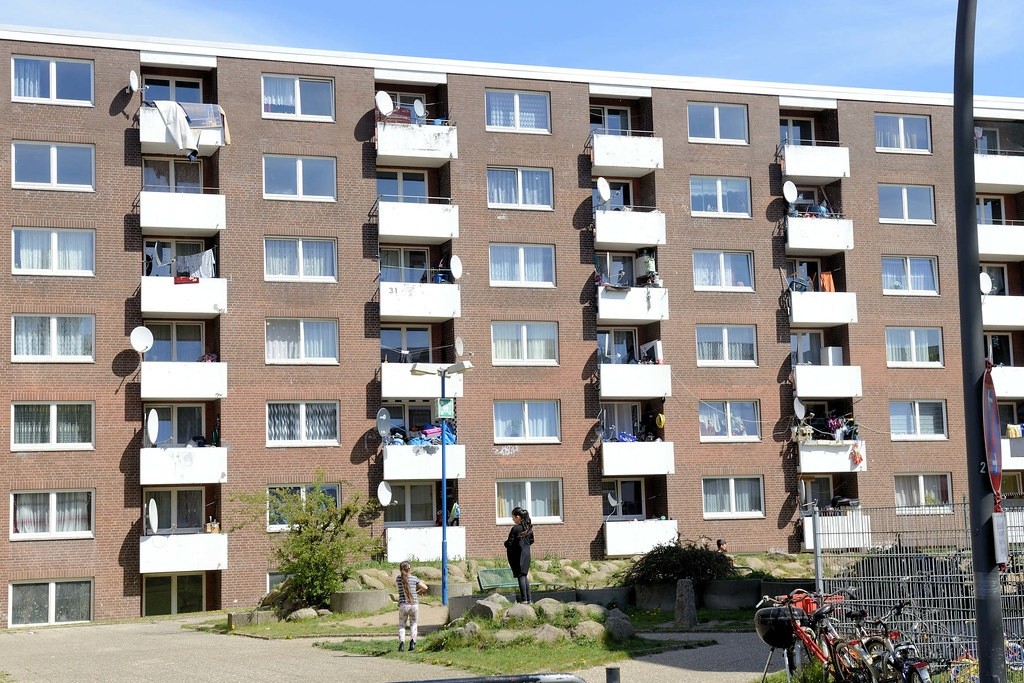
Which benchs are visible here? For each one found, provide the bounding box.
[477,568,542,593]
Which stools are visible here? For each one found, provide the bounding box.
[434,274,449,284]
[545,582,568,592]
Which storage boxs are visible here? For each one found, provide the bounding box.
[422,428,442,439]
[204,523,220,533]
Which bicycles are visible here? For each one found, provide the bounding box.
[754,585,931,683]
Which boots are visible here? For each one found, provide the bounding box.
[398,643,404,651]
[408,639,416,651]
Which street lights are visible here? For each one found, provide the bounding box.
[411,362,477,608]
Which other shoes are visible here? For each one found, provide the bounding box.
[521,600,531,604]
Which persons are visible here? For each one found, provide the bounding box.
[716,540,727,554]
[503,507,534,603]
[396,561,428,651]
